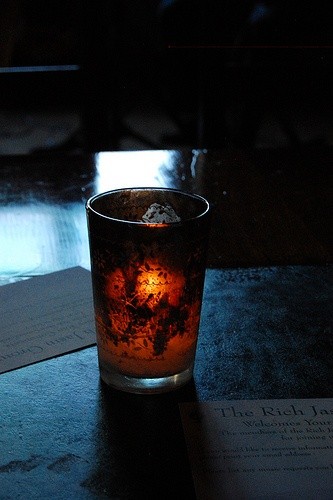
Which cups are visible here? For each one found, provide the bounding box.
[86,188,209,395]
